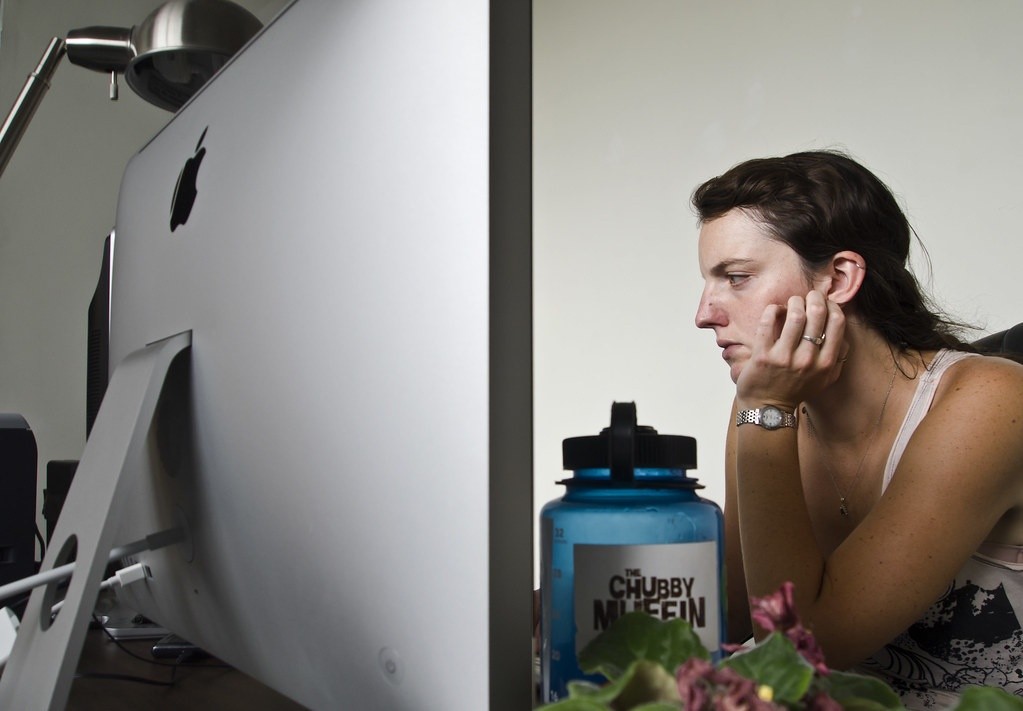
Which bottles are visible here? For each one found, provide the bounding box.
[539,401,728,706]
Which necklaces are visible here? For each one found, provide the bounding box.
[802,348,899,515]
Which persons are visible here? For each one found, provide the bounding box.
[533,152,1023,711]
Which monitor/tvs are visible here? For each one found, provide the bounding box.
[0,0,534,711]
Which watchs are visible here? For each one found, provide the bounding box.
[734,406,796,431]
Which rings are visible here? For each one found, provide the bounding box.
[804,334,825,346]
[837,355,848,364]
